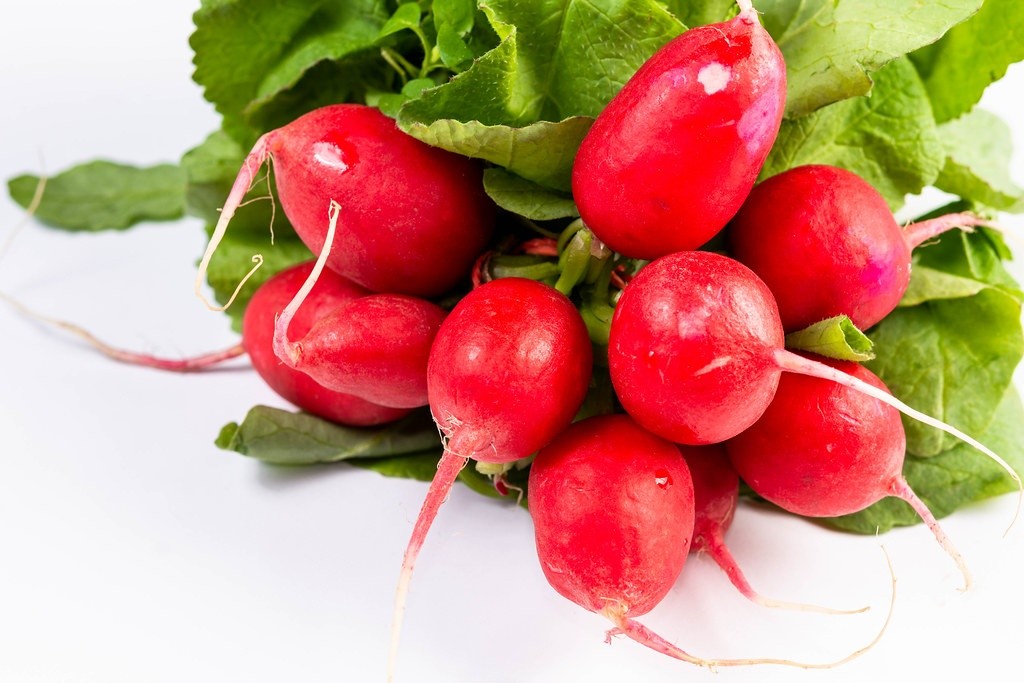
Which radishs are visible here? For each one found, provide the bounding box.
[0,0,1020,673]
